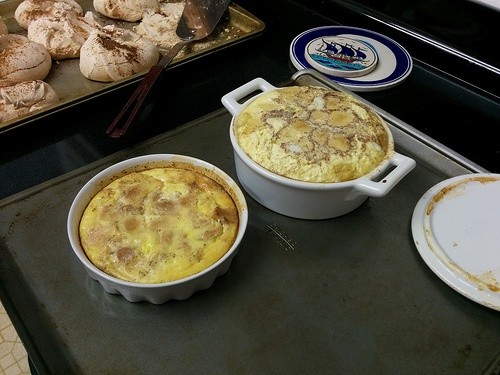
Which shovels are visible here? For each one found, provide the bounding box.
[102,0,231,140]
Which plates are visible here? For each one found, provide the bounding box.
[411,173,500,311]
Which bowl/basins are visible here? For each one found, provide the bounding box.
[67,153,249,305]
[222,77,415,220]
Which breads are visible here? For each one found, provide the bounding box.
[234,85,388,183]
[0,0,189,127]
[79,167,237,285]
